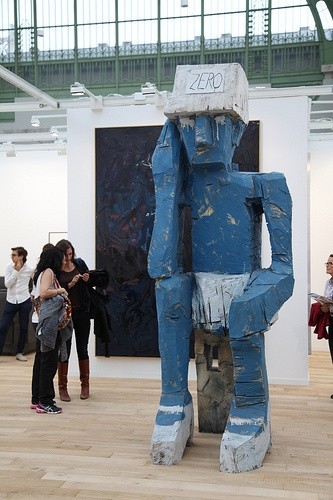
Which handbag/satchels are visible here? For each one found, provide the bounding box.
[32,269,72,331]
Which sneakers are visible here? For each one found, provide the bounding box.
[31,400,39,409]
[36,401,63,414]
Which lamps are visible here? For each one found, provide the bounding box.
[141,82,169,105]
[70,82,103,110]
[31,114,67,136]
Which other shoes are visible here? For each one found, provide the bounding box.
[16,353,28,362]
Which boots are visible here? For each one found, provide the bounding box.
[79,357,89,399]
[58,361,71,402]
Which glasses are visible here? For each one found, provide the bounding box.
[324,262,333,267]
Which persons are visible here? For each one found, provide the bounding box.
[149,63,295,472]
[31,243,68,414]
[0,246,34,361]
[320,254,333,399]
[56,239,92,402]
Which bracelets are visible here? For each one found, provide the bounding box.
[56,289,59,295]
